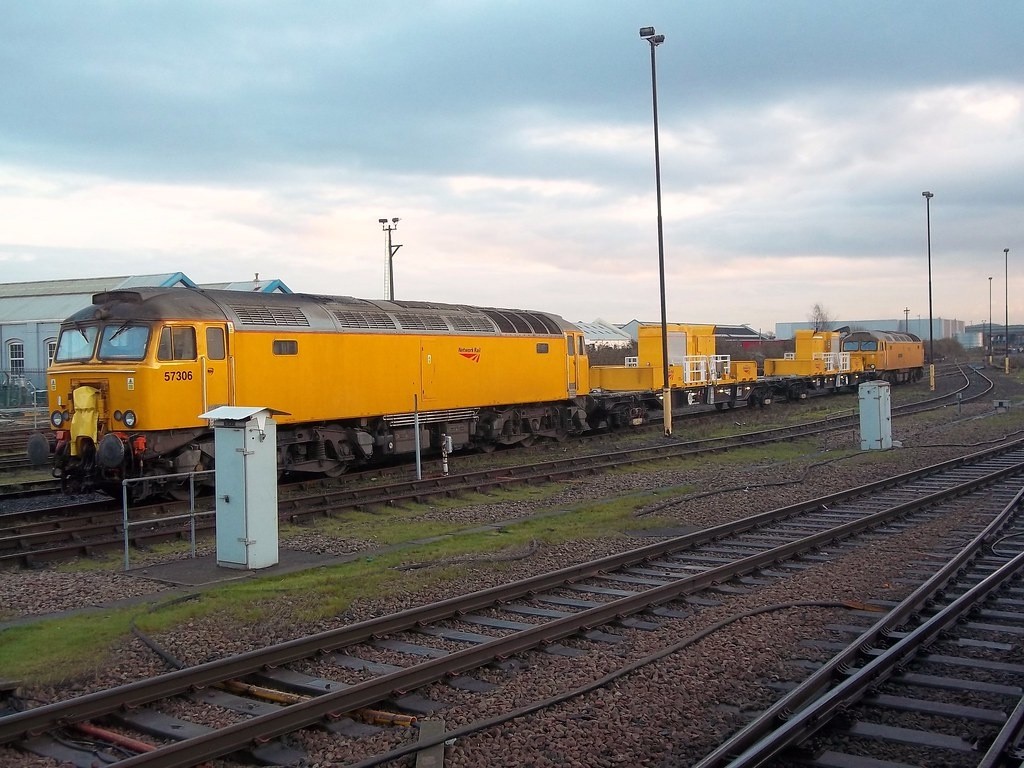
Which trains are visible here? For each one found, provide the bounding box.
[28,286,925,509]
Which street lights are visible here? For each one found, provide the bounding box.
[921,190,936,392]
[638,26,675,436]
[378,217,403,302]
